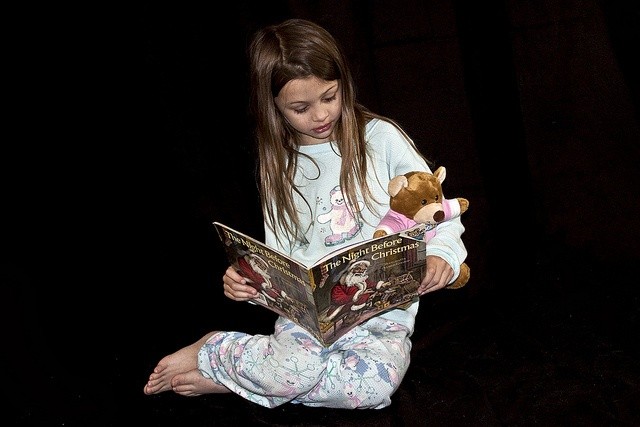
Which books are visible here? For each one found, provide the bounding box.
[212,222,427,348]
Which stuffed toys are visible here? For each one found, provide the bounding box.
[373,167,471,288]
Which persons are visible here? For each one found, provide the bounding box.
[143,18,467,411]
